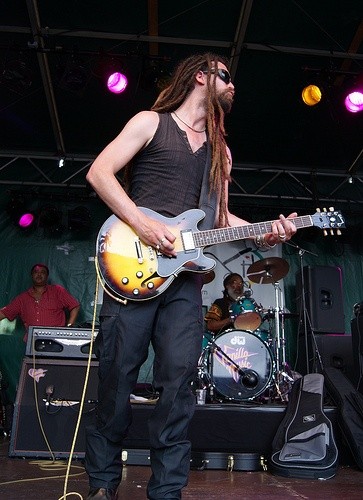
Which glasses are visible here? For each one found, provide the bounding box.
[228,280,244,286]
[203,69,235,85]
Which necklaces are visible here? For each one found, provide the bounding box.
[173,110,207,133]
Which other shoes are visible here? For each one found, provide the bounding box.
[87,485,118,500]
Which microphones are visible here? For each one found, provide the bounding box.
[242,373,258,388]
[45,384,54,406]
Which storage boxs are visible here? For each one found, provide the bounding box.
[9,356,99,459]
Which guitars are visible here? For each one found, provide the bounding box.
[95,206,345,301]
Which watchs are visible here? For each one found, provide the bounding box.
[67,323,72,326]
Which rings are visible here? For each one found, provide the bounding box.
[279,234,286,239]
[156,244,160,250]
[161,238,165,242]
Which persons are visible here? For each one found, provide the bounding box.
[81,54,300,500]
[205,272,267,332]
[0,263,81,342]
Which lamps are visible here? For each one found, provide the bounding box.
[329,76,363,129]
[295,64,336,111]
[96,57,130,96]
[7,202,37,230]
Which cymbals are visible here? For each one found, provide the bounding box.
[246,257,290,284]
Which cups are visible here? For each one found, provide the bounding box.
[196,390,206,404]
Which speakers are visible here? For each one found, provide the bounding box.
[296,331,357,399]
[8,359,100,457]
[294,265,346,334]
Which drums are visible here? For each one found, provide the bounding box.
[228,296,263,333]
[201,328,276,402]
